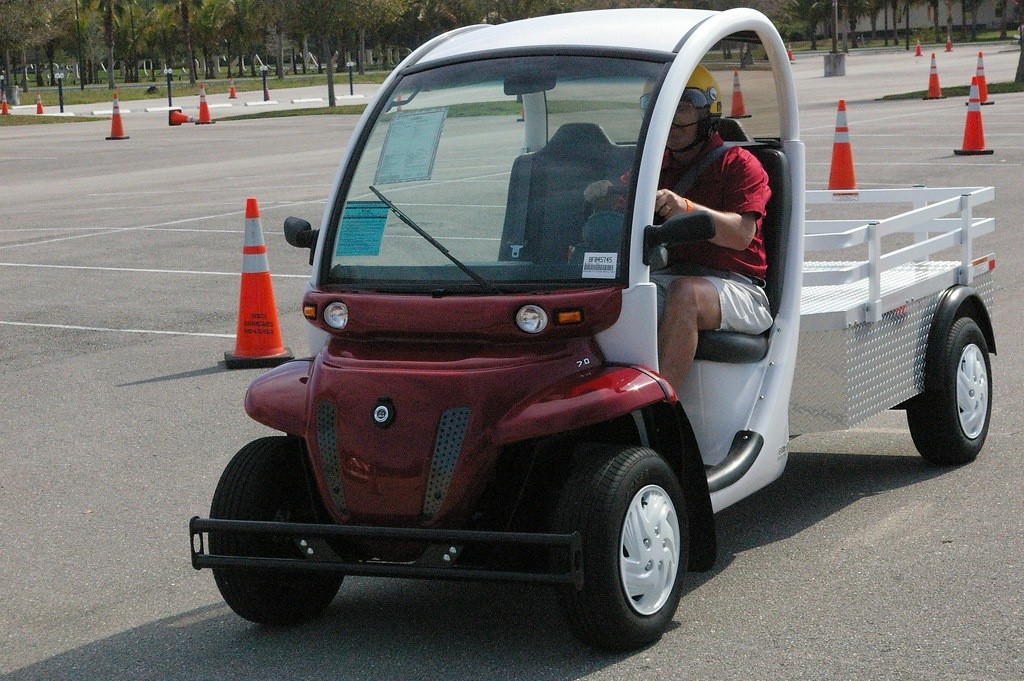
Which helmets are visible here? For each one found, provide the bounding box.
[641,61,723,121]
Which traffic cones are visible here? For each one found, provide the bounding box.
[827,100,862,198]
[227,76,238,100]
[0,90,12,115]
[168,109,200,126]
[396,94,403,113]
[724,71,752,120]
[953,76,995,156]
[922,53,947,100]
[965,50,995,106]
[914,38,923,56]
[516,103,524,122]
[786,42,795,61]
[195,82,216,125]
[944,32,954,52]
[105,91,130,140]
[36,88,45,115]
[223,195,295,369]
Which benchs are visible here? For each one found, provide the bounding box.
[497,121,793,364]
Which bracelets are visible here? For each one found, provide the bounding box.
[684,198,694,213]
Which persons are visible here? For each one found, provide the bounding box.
[585,62,773,392]
